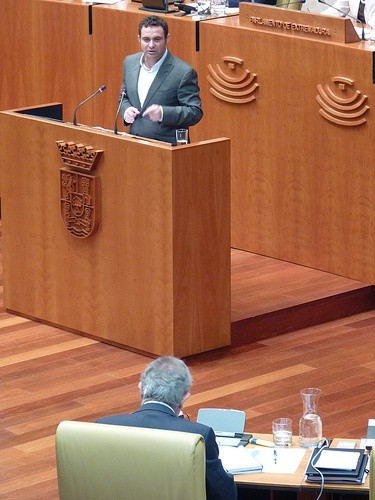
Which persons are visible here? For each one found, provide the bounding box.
[94,356,238,500]
[224,0,375,41]
[118,15,204,146]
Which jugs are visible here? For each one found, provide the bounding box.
[298,387,322,448]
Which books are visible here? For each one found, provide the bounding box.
[304,447,370,485]
[219,445,264,475]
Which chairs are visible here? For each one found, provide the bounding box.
[55,420,207,500]
[196,408,246,433]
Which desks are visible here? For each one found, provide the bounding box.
[222,432,375,500]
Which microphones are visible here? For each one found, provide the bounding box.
[72,86,106,127]
[317,0,364,40]
[273,0,305,7]
[113,88,127,134]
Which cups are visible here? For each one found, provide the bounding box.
[210,0,225,17]
[176,129,188,146]
[272,418,292,446]
